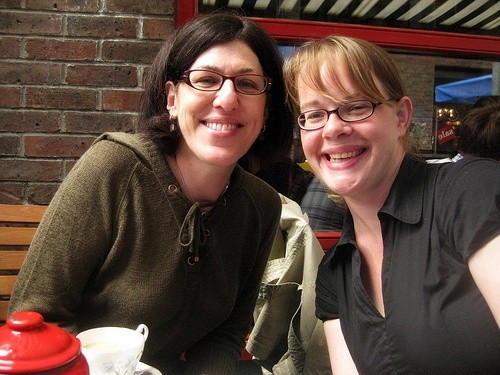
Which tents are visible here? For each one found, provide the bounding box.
[434,75,494,160]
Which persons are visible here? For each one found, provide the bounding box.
[253,116,347,231]
[4,12,282,375]
[280,36,499,375]
[455,94,499,164]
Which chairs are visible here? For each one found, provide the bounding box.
[0,204,49,326]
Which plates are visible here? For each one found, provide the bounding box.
[133,361,162,375]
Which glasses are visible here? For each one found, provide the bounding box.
[297,96,400,130]
[178,69,273,95]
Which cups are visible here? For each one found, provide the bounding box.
[75,324,150,375]
